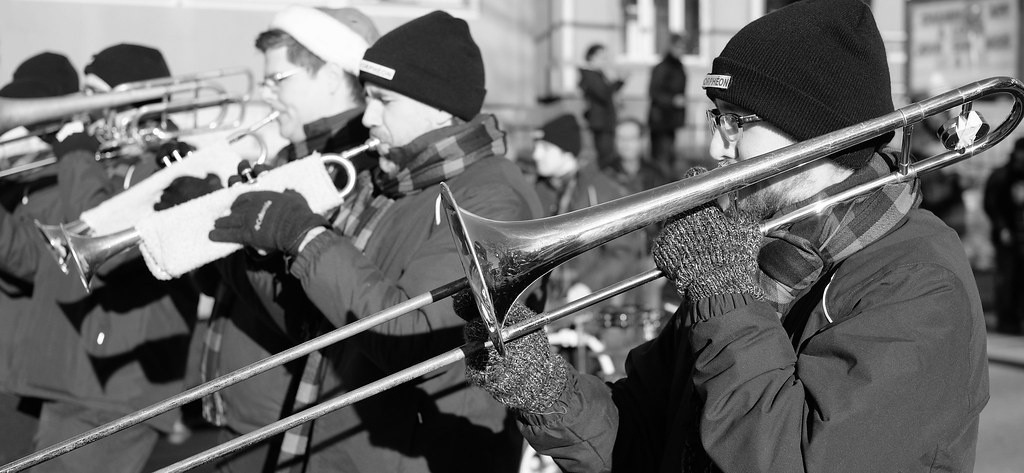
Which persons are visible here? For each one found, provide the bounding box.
[1,41,204,472]
[2,49,86,470]
[452,0,995,471]
[140,3,379,472]
[513,109,640,355]
[899,90,1024,339]
[644,33,689,171]
[602,116,672,345]
[153,4,552,472]
[575,40,627,170]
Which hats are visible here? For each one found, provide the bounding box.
[702,1,895,170]
[0,53,79,100]
[532,115,581,155]
[84,43,172,122]
[357,10,488,121]
[266,5,382,78]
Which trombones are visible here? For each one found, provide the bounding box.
[0,66,268,179]
[0,76,1024,473]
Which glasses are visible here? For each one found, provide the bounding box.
[256,65,306,94]
[706,108,765,144]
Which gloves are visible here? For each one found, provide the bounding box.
[52,120,103,162]
[451,289,569,413]
[209,188,334,260]
[155,139,198,170]
[228,157,271,186]
[153,172,224,211]
[648,166,766,302]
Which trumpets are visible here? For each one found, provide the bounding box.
[33,109,287,278]
[60,133,381,288]
